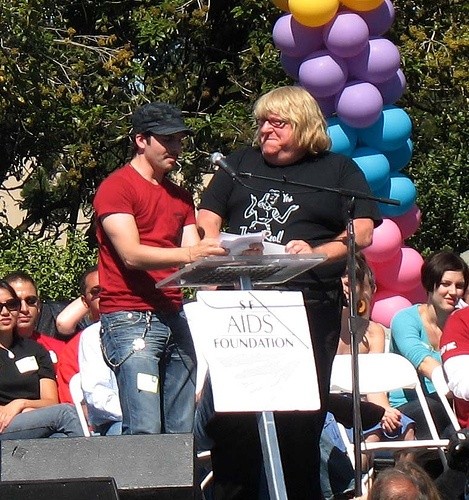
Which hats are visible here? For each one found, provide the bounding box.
[132,102,195,142]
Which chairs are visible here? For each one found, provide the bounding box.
[329,353,469,496]
[69,372,91,437]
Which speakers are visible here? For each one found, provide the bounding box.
[0,434,194,500]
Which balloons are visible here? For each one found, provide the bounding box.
[268,0,430,358]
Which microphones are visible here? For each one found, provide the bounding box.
[210,152,246,187]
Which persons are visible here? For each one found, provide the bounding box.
[366,457,442,500]
[442,266,469,439]
[95,102,233,441]
[53,253,441,500]
[188,83,384,500]
[393,249,469,460]
[0,280,89,439]
[1,271,80,440]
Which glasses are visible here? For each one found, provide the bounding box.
[21,296,38,304]
[256,118,288,128]
[0,299,19,313]
[90,286,100,295]
